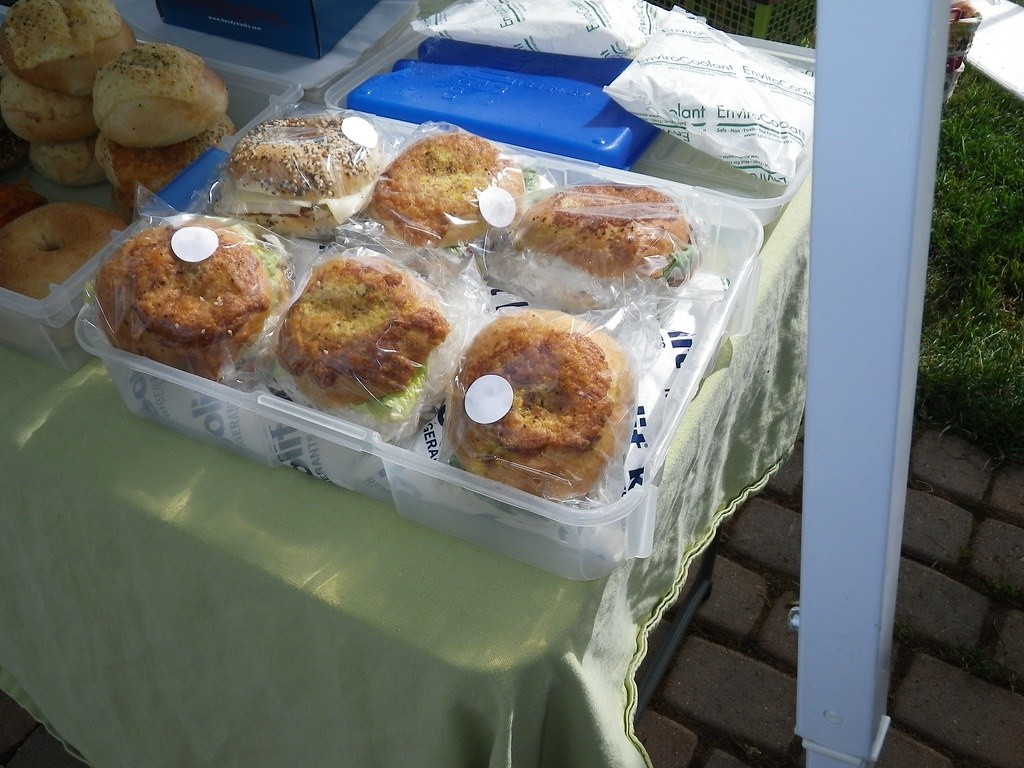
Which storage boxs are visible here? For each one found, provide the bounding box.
[0,4,304,372]
[155,0,381,60]
[323,30,811,227]
[74,97,766,582]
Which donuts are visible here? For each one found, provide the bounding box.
[0,201,128,305]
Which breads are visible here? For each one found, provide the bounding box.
[93,115,704,502]
[0,0,238,222]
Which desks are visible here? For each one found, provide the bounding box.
[0,0,811,768]
